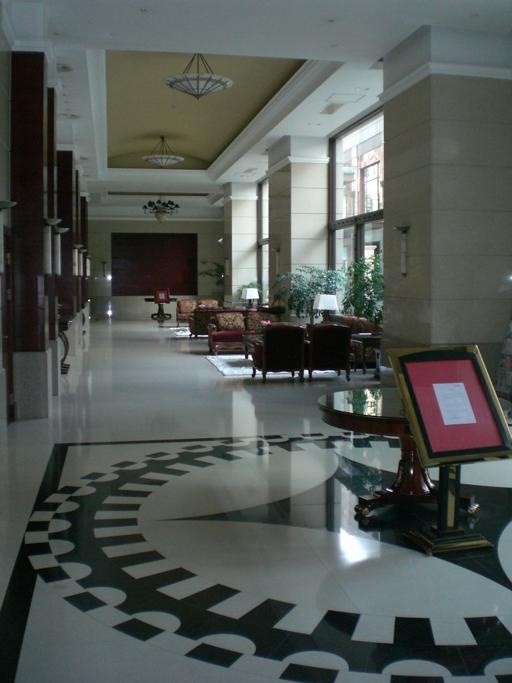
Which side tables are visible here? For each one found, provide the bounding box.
[353,334,381,373]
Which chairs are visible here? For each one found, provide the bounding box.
[177,297,352,385]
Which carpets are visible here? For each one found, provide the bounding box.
[169,326,208,338]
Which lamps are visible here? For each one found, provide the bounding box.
[311,292,339,322]
[141,135,185,168]
[240,286,260,308]
[271,245,281,276]
[165,53,234,101]
[143,194,180,224]
[393,222,411,276]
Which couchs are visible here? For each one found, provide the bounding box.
[330,313,382,369]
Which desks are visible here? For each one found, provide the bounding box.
[316,385,511,517]
[144,296,177,318]
